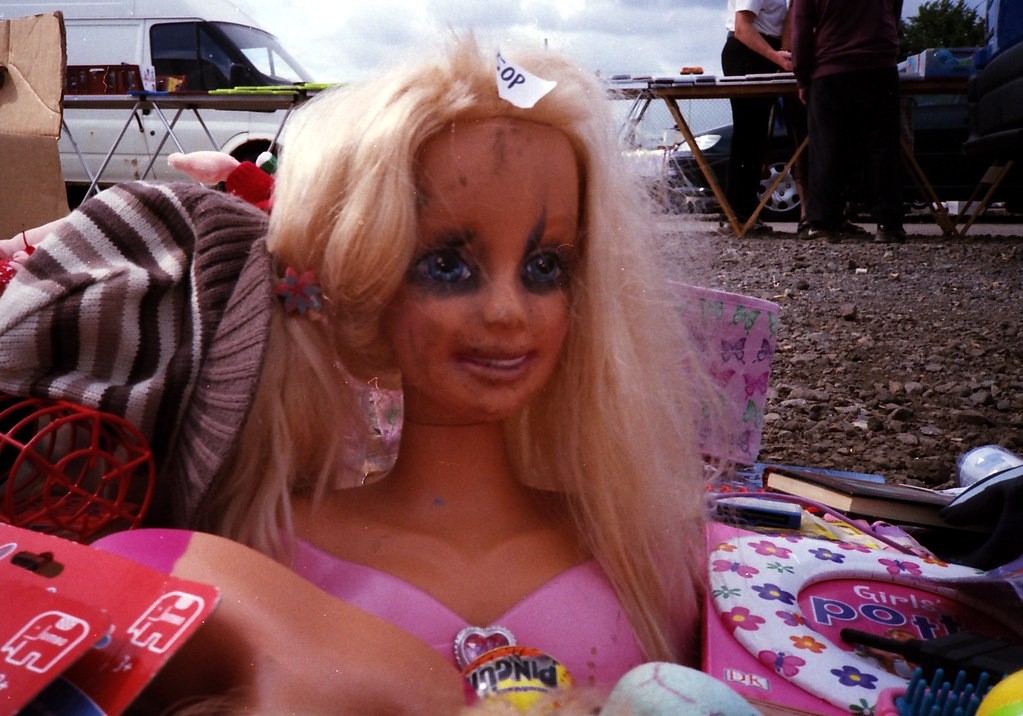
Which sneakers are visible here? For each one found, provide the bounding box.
[795,224,833,247]
[825,220,867,245]
[875,223,919,245]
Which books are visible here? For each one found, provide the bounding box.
[762,467,960,529]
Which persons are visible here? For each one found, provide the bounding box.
[81,14,716,715]
[721,1,911,239]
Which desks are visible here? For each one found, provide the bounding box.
[599,73,1023,242]
[61,90,311,217]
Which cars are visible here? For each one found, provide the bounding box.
[664,63,1023,219]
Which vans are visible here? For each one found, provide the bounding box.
[0,0,315,211]
